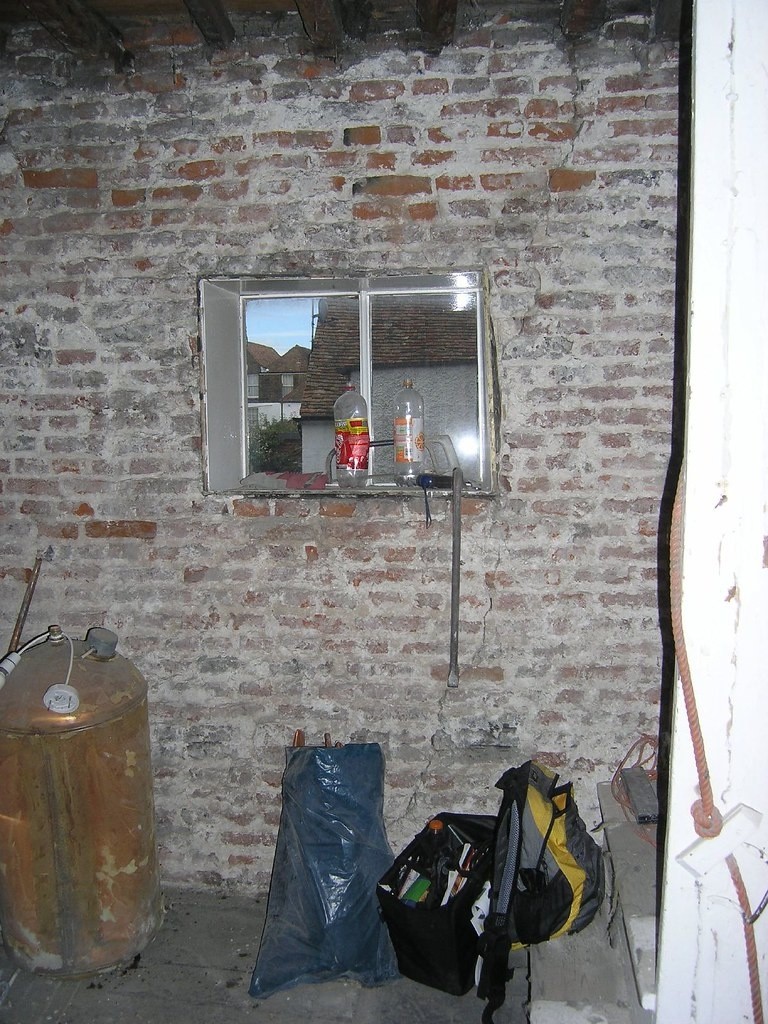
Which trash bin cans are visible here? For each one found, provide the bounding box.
[375,811,498,997]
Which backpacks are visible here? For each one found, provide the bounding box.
[477,760,605,999]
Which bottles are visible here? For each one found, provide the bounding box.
[392,378,425,488]
[333,382,370,487]
[420,820,445,850]
